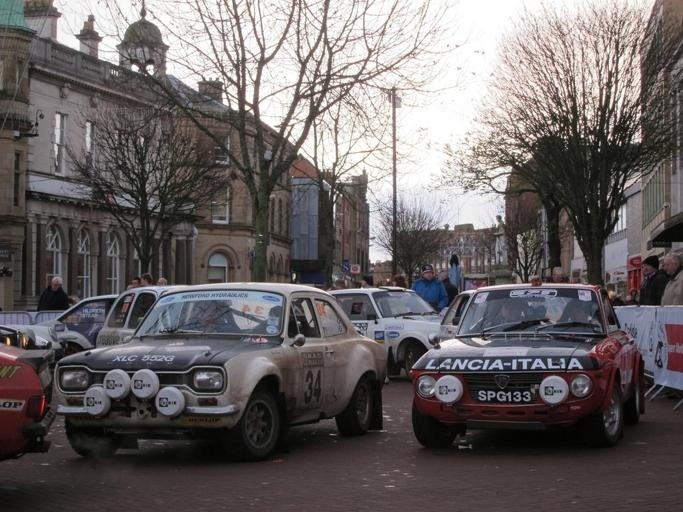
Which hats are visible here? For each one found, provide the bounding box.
[422,264,434,274]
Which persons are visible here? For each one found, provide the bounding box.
[438,272,459,306]
[549,266,568,284]
[334,280,344,290]
[559,306,600,332]
[186,302,220,330]
[608,251,683,398]
[493,301,523,332]
[126,274,169,291]
[411,263,449,310]
[37,275,68,310]
[360,277,373,289]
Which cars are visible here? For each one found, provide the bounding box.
[325,286,450,383]
[437,289,568,341]
[52,282,388,463]
[0,283,188,380]
[0,343,58,464]
[406,274,647,451]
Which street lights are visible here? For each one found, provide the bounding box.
[384,86,401,278]
[263,150,273,177]
